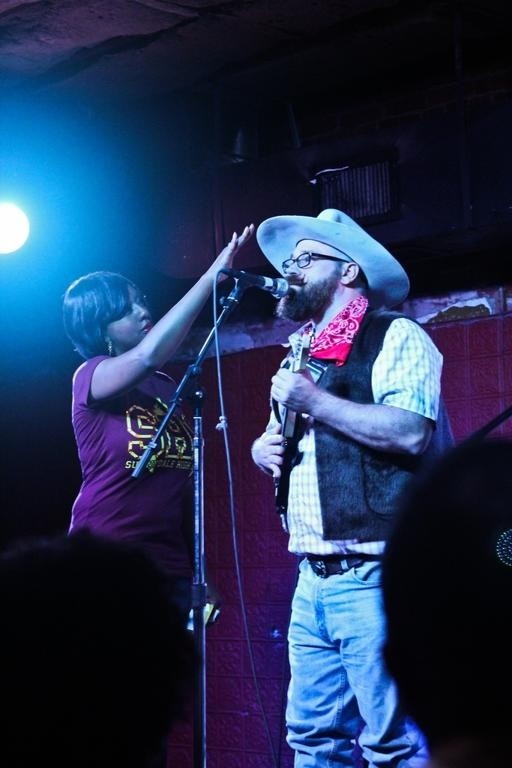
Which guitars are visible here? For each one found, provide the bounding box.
[274,320,315,518]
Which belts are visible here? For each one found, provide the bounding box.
[305,554,377,580]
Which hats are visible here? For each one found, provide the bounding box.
[256,209,411,309]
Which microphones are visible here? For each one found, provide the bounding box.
[221,268,290,300]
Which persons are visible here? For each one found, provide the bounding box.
[246,206,445,768]
[381,438,512,768]
[0,538,191,768]
[62,223,256,578]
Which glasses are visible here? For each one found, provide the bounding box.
[282,252,350,274]
